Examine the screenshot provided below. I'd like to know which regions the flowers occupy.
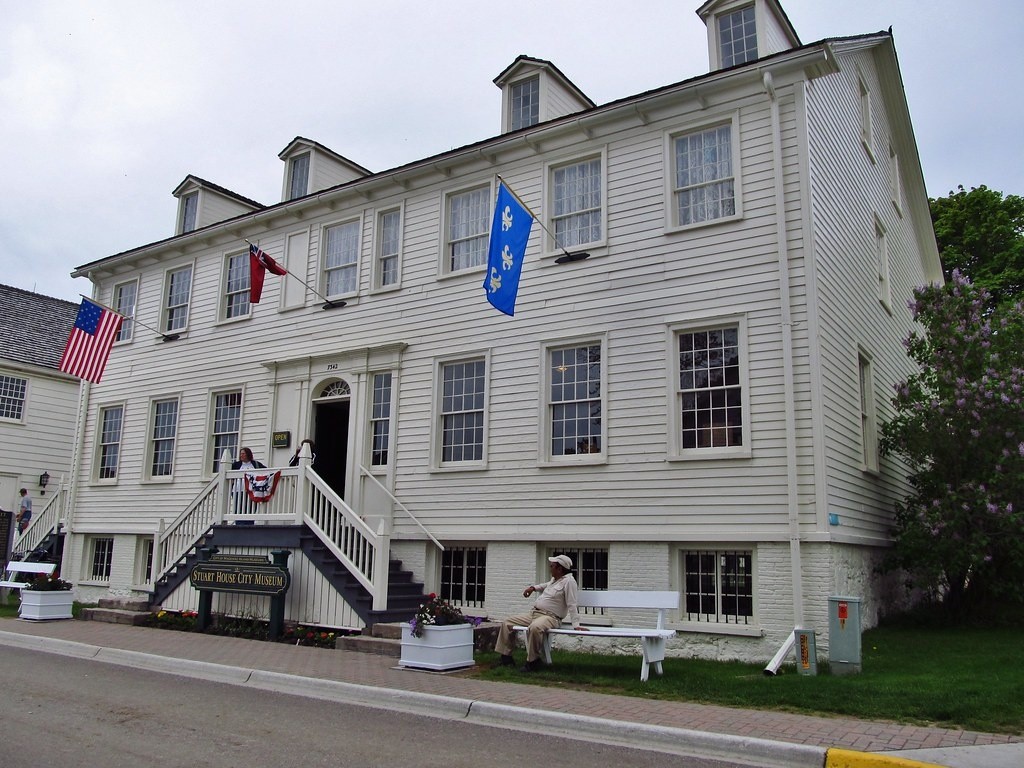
[409,592,483,640]
[24,572,74,591]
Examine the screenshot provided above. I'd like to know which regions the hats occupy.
[548,555,573,569]
[19,488,27,493]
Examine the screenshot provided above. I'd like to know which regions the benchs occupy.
[2,561,57,613]
[511,589,681,683]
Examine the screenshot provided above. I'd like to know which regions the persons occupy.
[15,488,32,535]
[19,518,29,533]
[229,447,270,524]
[488,553,590,673]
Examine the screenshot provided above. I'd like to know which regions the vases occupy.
[397,622,476,671]
[18,589,76,621]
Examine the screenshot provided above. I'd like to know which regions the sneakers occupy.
[519,657,543,672]
[501,654,515,668]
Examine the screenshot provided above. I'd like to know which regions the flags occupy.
[248,243,287,304]
[482,183,533,317]
[57,298,124,384]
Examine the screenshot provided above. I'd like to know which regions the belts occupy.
[533,606,563,620]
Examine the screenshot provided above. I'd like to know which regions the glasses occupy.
[549,564,558,568]
